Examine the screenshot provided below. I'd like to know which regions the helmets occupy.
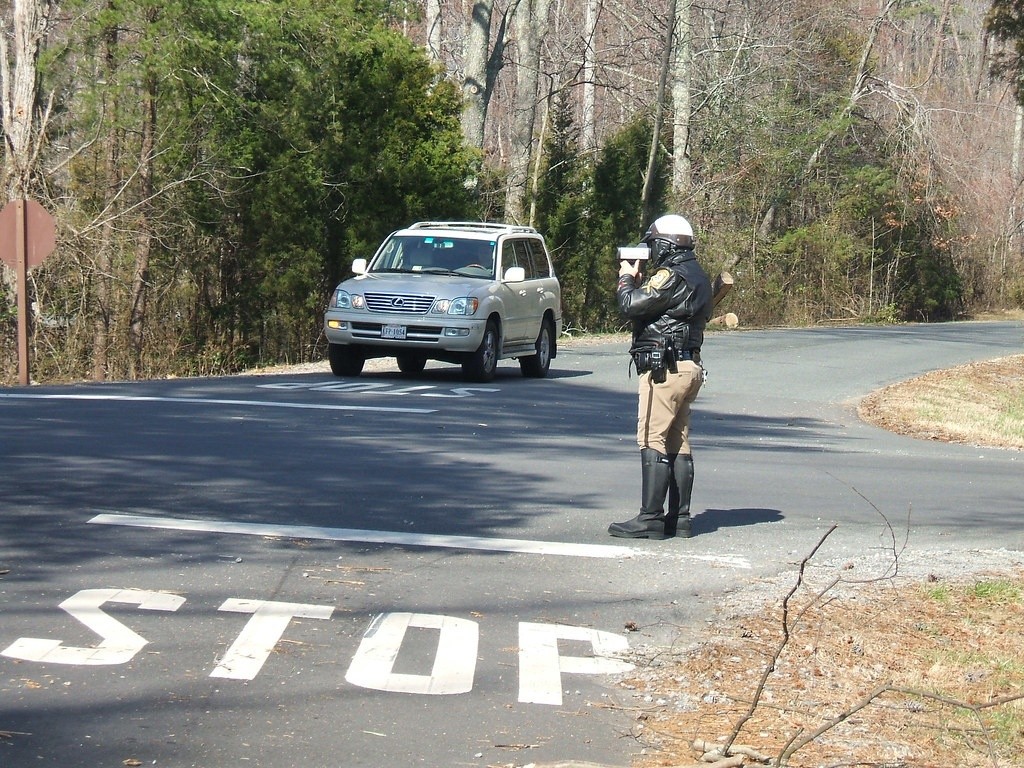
[637,215,697,251]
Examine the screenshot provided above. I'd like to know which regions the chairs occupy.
[403,248,434,270]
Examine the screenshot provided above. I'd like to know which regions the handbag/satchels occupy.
[628,331,680,385]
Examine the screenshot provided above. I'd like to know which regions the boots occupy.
[665,452,694,538]
[607,447,671,540]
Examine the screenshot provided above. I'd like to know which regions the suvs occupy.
[324,222,562,383]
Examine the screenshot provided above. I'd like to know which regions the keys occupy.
[701,369,708,388]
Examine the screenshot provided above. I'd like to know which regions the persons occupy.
[607,214,713,540]
